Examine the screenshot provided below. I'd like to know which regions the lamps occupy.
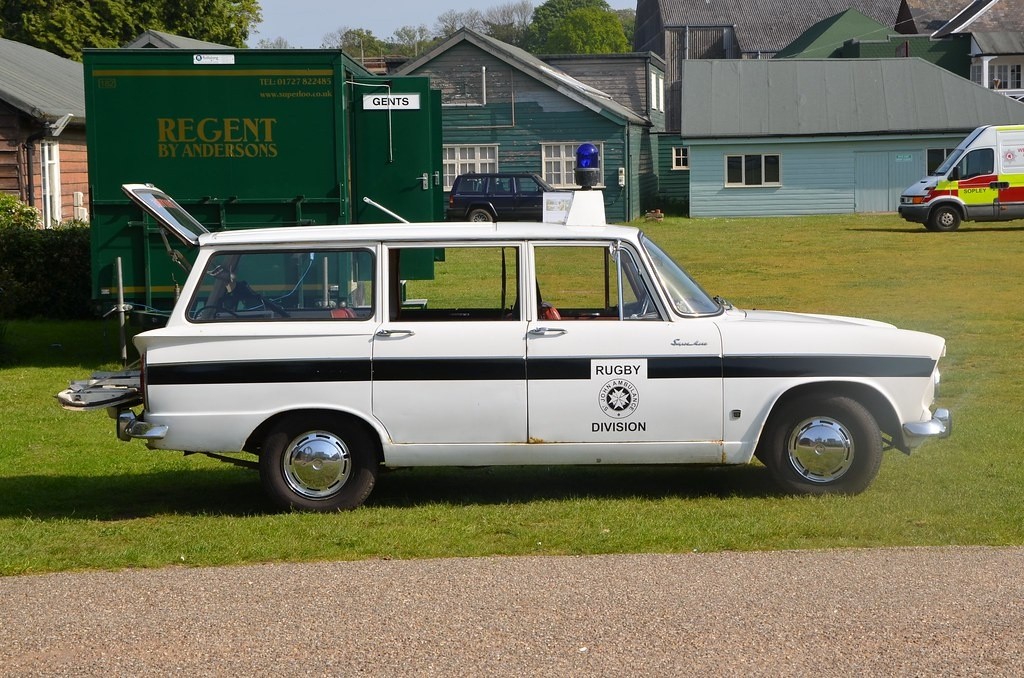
[49,113,75,137]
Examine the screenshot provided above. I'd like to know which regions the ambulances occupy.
[898,124,1024,231]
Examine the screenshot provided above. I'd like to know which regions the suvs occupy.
[447,170,566,221]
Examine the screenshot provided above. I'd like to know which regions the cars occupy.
[54,143,952,514]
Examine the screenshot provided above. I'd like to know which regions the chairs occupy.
[340,308,357,319]
[331,309,348,318]
[463,179,518,194]
[400,280,428,309]
[536,277,561,319]
